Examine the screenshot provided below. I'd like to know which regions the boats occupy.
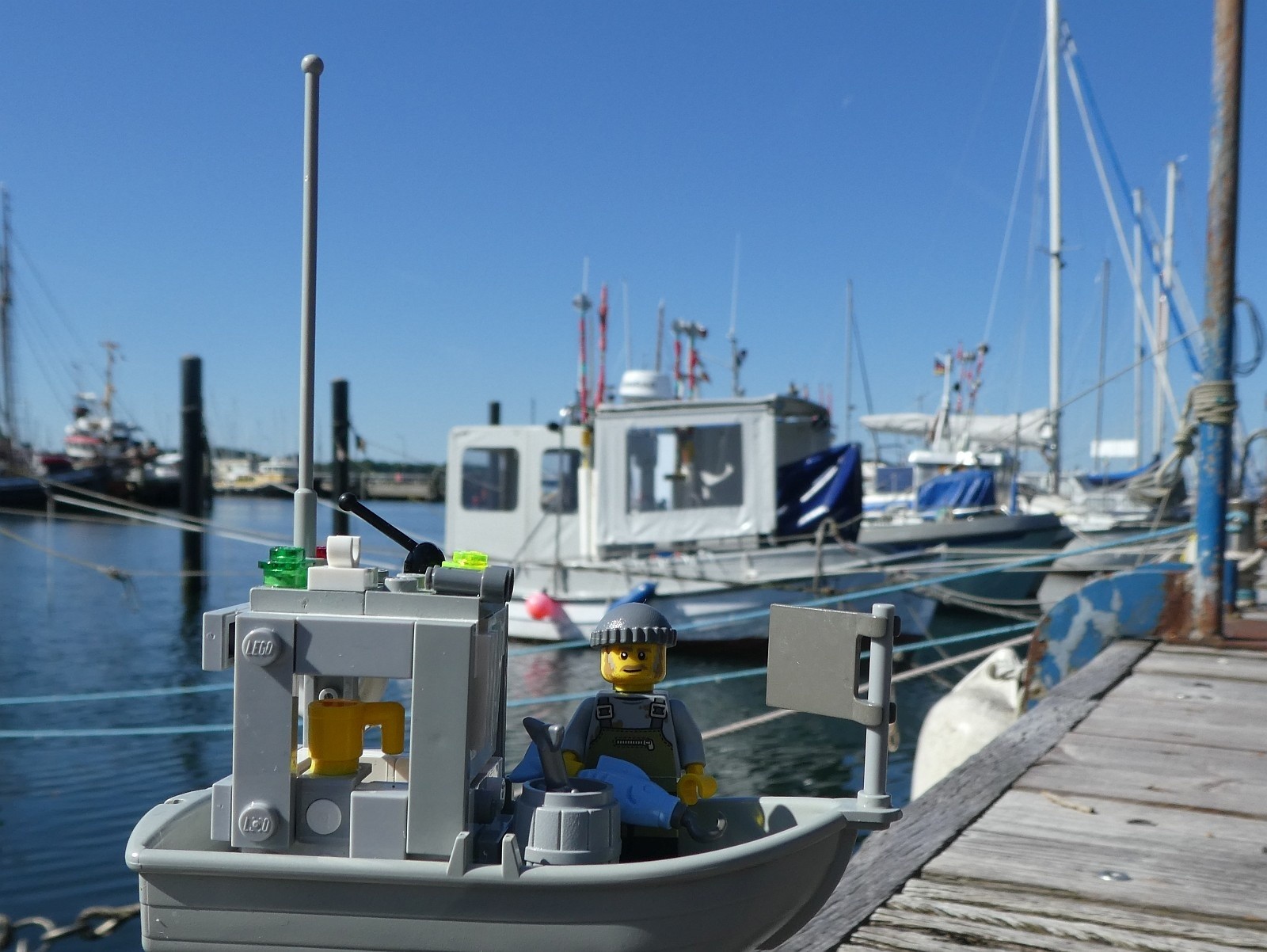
[424,265,1062,656]
[120,38,901,949]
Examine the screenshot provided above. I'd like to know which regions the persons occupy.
[561,602,718,840]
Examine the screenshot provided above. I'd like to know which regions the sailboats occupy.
[857,0,1251,611]
[0,200,182,515]
